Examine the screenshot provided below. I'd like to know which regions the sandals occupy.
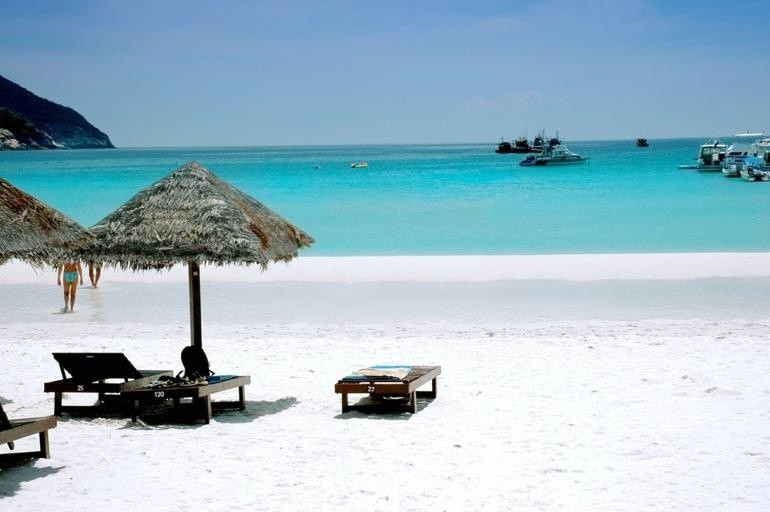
[162,371,208,387]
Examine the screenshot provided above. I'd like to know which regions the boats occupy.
[497,134,558,154]
[520,144,587,167]
[637,138,649,147]
[679,139,770,181]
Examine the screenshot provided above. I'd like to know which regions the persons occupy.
[88,261,103,288]
[57,257,83,313]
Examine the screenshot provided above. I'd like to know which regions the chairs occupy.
[0,404,57,458]
[44,353,173,417]
[335,364,442,414]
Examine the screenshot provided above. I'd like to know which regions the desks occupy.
[120,375,250,423]
